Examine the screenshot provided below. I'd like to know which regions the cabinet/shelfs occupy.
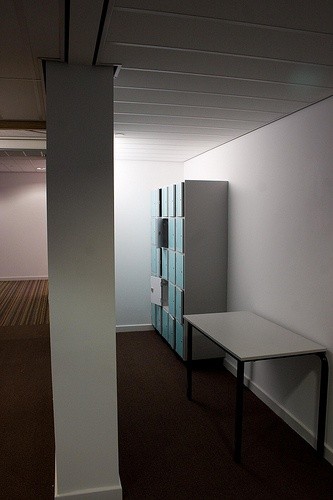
[150,180,228,368]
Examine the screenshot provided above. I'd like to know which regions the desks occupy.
[181,311,329,465]
